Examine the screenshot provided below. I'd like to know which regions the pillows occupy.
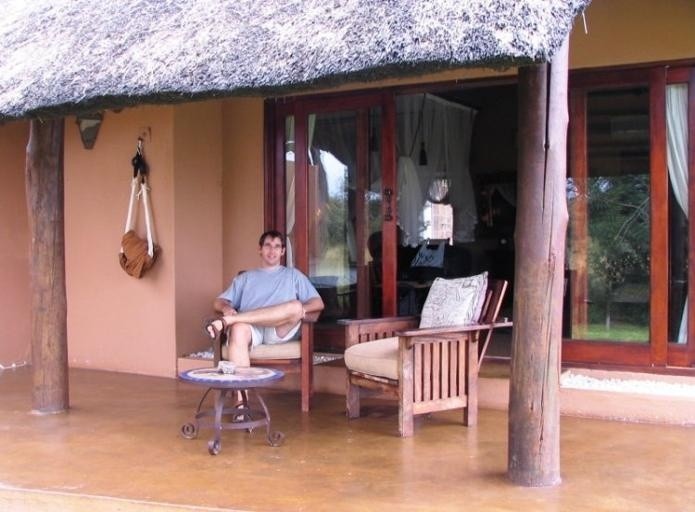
[417,272,489,330]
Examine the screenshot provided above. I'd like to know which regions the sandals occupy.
[232,401,255,431]
[203,314,227,339]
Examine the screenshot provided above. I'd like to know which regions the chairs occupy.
[208,314,315,415]
[338,271,512,440]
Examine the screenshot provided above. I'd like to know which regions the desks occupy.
[179,367,283,453]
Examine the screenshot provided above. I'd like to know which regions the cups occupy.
[223,365,234,373]
[218,361,229,372]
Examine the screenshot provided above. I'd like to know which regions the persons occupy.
[206,231,325,420]
